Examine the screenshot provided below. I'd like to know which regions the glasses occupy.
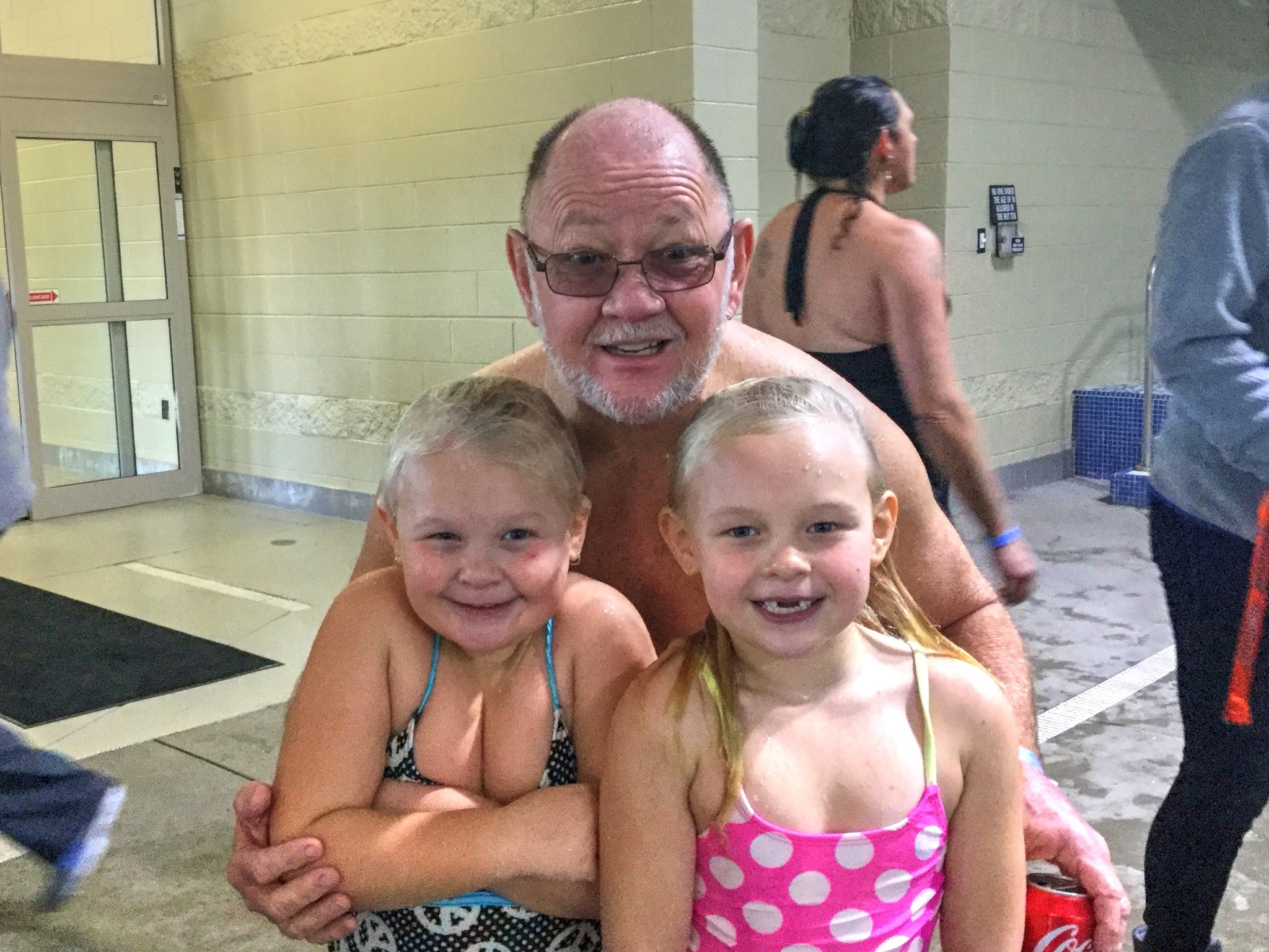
[524,218,736,297]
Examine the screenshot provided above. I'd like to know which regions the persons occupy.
[226,99,1130,952]
[0,291,125,911]
[1134,90,1268,952]
[744,76,1043,609]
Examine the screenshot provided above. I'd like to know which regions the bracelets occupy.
[989,526,1022,552]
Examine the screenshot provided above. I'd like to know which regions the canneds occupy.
[1021,872,1096,952]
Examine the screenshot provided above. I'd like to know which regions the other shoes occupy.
[43,773,127,910]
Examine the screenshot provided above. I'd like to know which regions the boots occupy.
[1132,923,1222,952]
